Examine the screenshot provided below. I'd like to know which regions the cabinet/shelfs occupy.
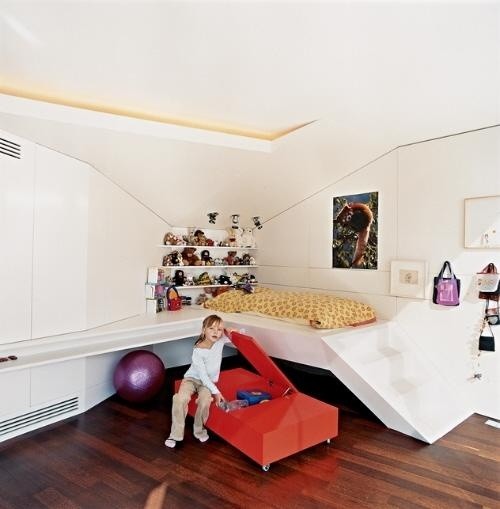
[175,331,338,472]
[159,245,258,301]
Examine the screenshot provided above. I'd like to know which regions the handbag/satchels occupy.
[476,263,500,292]
[437,263,459,306]
[479,318,495,352]
[480,261,500,295]
[486,314,500,326]
[434,261,460,303]
[479,267,500,301]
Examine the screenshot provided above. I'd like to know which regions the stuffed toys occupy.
[153,226,259,313]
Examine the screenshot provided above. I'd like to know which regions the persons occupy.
[164,315,245,449]
